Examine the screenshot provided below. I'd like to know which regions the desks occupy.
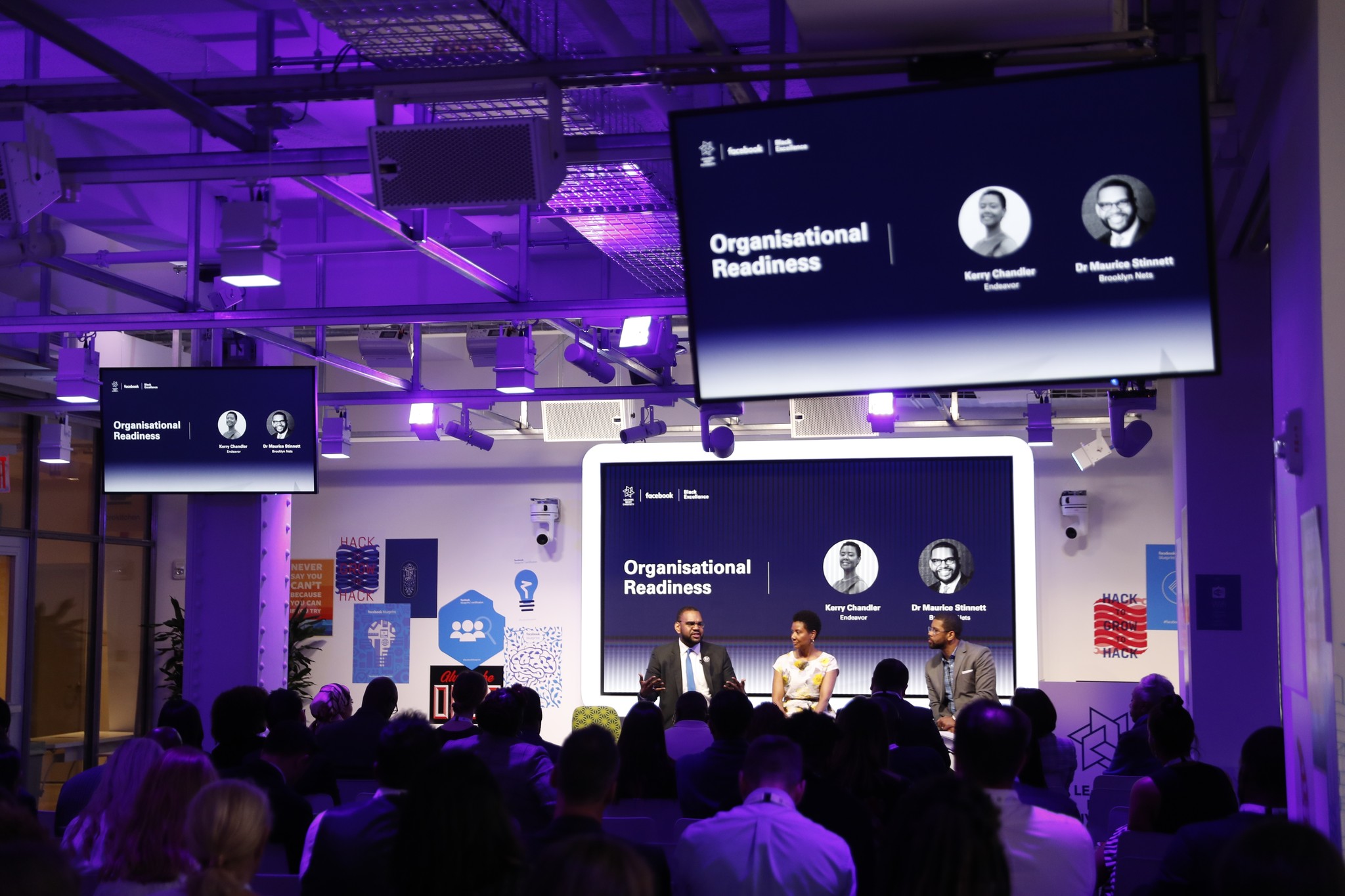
[32,729,133,795]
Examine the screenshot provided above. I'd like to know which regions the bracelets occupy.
[952,715,956,722]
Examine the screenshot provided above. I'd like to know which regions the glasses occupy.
[930,557,960,565]
[1097,198,1131,211]
[302,709,306,714]
[391,703,398,714]
[676,620,705,628]
[927,626,950,635]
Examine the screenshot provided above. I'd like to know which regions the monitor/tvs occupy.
[100,365,320,495]
[669,48,1224,406]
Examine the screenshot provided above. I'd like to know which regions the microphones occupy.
[700,659,704,664]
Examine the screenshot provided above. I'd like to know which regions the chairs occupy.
[608,815,657,847]
[251,778,378,896]
[1015,772,1180,896]
[571,707,622,743]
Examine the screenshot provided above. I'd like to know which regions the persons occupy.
[924,612,1000,734]
[0,669,1345,896]
[222,412,241,439]
[772,611,839,718]
[972,189,1021,258]
[926,542,972,594]
[833,542,869,594]
[637,607,747,729]
[1096,179,1152,254]
[271,412,293,439]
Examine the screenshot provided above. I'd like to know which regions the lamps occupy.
[1022,389,1057,447]
[619,405,667,444]
[1071,429,1113,470]
[491,323,538,396]
[618,314,679,371]
[563,321,615,384]
[218,181,285,287]
[37,412,74,465]
[407,403,443,442]
[698,401,744,458]
[444,406,496,453]
[1109,391,1157,457]
[53,333,101,404]
[320,403,352,460]
[867,392,900,435]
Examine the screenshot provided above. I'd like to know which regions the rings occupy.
[941,727,944,729]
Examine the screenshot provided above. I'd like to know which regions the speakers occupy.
[367,114,569,211]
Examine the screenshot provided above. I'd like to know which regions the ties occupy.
[686,649,696,692]
